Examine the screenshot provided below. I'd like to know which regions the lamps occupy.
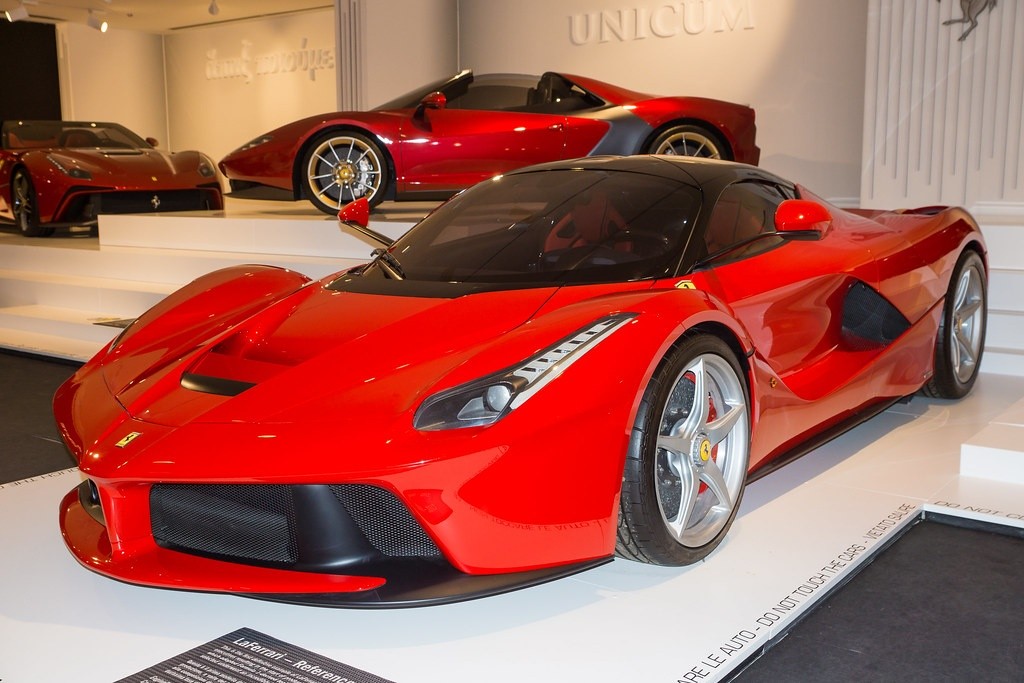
[5,3,30,23]
[87,14,109,33]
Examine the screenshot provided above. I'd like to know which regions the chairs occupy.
[704,201,771,260]
[539,196,634,256]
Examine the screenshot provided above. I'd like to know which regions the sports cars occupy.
[218,69,760,215]
[53,154,989,609]
[0,120,225,236]
